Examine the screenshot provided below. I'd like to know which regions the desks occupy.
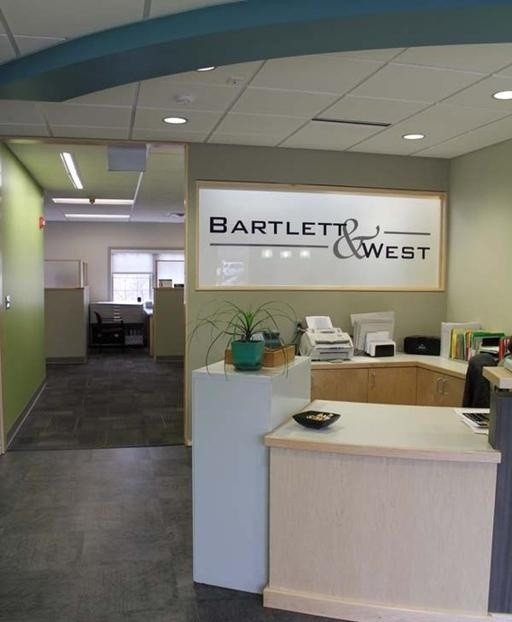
[143,308,154,356]
[262,398,502,621]
[481,365,511,621]
[191,353,312,595]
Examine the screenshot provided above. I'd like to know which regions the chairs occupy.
[93,310,125,354]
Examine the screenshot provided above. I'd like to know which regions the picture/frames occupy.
[193,179,448,291]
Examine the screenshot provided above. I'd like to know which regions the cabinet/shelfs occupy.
[311,369,368,405]
[416,367,466,404]
[44,286,89,365]
[152,286,185,360]
[368,367,416,405]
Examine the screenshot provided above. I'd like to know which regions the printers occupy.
[298,315,354,360]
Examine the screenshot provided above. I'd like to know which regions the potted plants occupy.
[186,297,305,381]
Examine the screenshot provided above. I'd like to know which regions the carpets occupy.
[4,352,184,452]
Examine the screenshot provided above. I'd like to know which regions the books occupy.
[451,328,512,362]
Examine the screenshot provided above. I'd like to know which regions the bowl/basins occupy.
[291,410,341,429]
